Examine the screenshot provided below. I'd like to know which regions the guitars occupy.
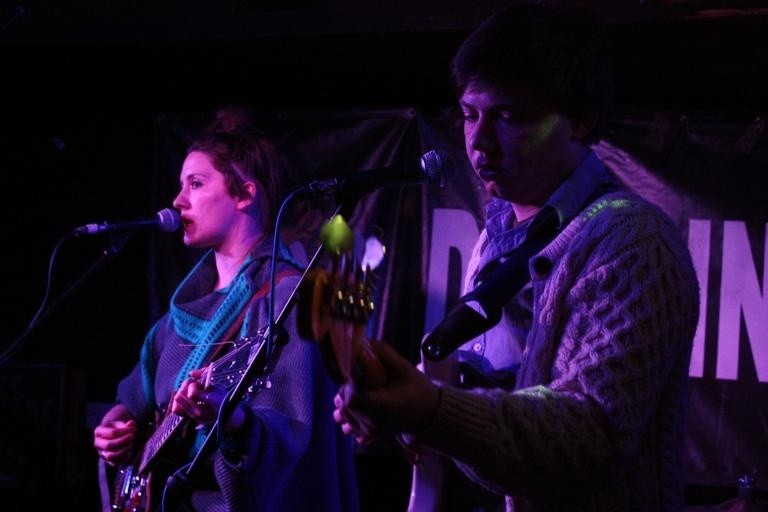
[109,323,286,511]
[296,254,458,512]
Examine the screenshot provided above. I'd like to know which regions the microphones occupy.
[293,148,453,204]
[76,206,182,238]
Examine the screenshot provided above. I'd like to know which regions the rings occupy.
[195,391,209,409]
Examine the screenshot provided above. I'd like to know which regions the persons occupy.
[89,103,364,511]
[331,10,704,510]
